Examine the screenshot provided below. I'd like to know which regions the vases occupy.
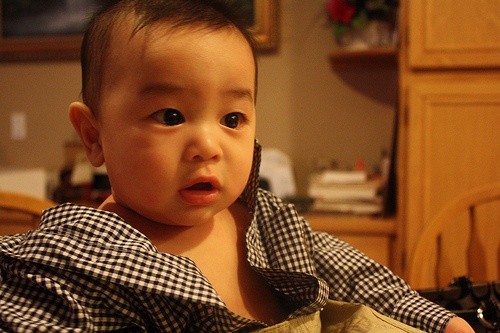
[346,18,389,48]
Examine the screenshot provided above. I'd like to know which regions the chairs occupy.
[407,182,500,290]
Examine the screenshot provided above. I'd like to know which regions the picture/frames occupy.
[0,0,280,63]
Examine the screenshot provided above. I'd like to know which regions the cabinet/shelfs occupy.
[398,0,500,290]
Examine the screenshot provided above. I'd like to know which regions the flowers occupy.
[322,0,376,48]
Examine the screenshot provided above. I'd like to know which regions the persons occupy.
[1,0,476,333]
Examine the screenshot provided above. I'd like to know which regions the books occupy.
[309,168,383,215]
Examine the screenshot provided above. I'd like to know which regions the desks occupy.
[52,194,396,285]
